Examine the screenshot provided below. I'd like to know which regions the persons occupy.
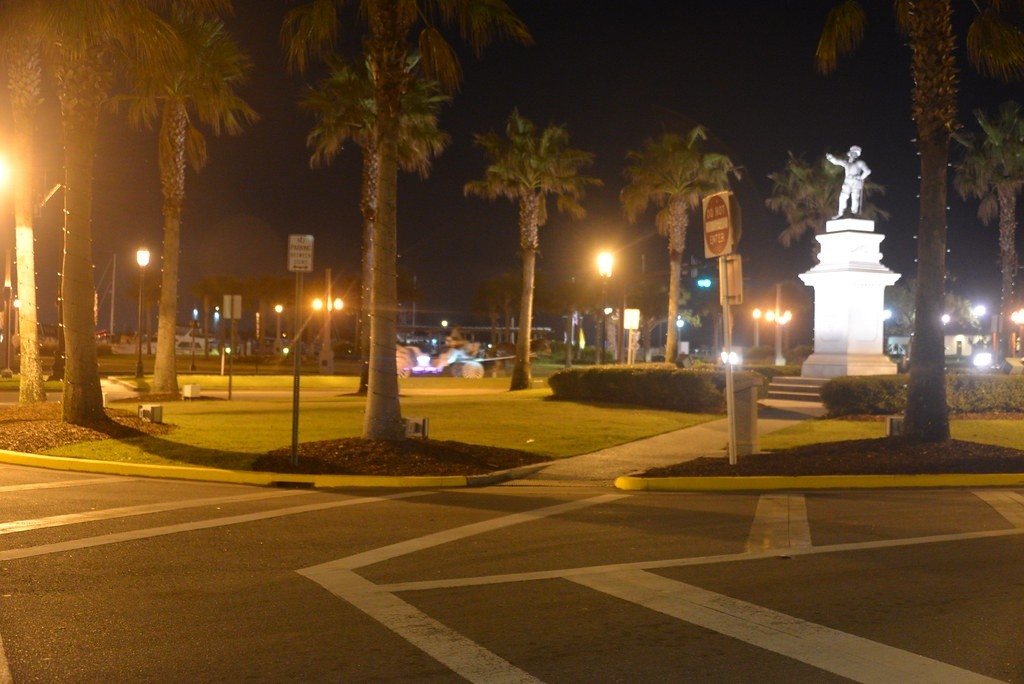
[825,145,871,220]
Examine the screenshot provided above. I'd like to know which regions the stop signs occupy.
[701,191,734,258]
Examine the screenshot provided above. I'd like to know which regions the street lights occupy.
[275,300,284,355]
[312,295,348,376]
[596,248,617,365]
[750,301,1024,381]
[441,319,448,352]
[190,304,198,374]
[135,244,151,379]
[212,303,220,358]
